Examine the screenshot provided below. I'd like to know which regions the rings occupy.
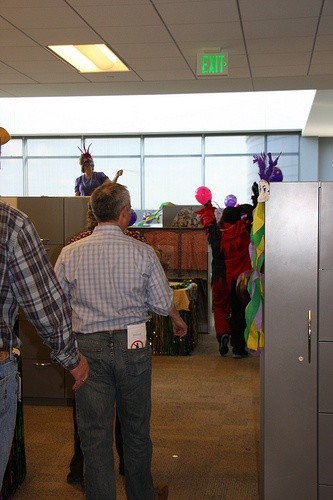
[82,376,87,382]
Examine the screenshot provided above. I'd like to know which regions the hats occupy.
[81,154,92,173]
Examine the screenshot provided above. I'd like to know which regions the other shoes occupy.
[154,485,169,500]
[66,471,87,491]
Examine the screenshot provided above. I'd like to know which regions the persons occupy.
[64,198,188,488]
[198,181,260,361]
[74,153,123,197]
[0,126,91,491]
[52,180,176,499]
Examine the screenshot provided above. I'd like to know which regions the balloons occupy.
[223,194,237,209]
[264,166,284,182]
[127,209,137,226]
[194,186,213,205]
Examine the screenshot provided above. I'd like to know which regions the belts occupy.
[0,348,21,361]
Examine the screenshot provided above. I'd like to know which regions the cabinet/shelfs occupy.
[127,228,210,281]
[2,195,95,407]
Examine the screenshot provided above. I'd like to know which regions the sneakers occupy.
[220,333,230,354]
[232,347,249,359]
